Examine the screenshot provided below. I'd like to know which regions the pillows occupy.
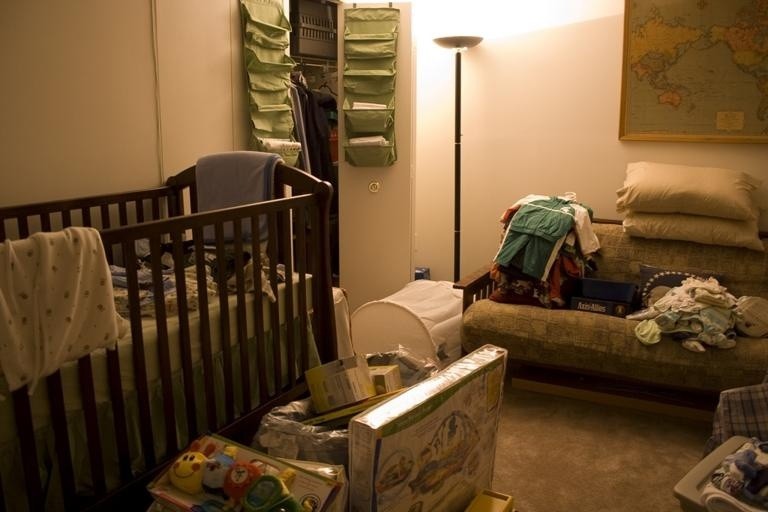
[617,161,765,252]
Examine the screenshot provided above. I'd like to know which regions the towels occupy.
[194,151,284,246]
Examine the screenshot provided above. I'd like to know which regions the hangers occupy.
[317,64,337,96]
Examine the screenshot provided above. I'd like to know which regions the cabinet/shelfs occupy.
[230,0,414,316]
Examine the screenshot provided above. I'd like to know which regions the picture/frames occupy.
[617,0,767,146]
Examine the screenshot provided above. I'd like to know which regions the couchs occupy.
[453,218,768,389]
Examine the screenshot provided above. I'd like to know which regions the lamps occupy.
[434,36,482,283]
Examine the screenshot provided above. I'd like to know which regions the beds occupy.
[1,150,338,511]
